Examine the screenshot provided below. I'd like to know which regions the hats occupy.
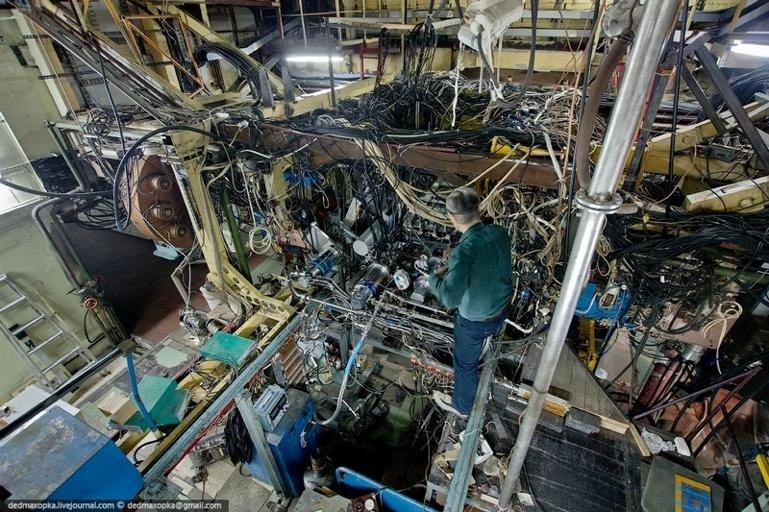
[446,187,479,215]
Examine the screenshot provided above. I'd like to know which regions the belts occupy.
[488,304,509,322]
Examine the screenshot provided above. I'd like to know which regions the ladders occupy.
[0,274,110,392]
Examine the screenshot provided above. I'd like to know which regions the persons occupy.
[429,188,511,418]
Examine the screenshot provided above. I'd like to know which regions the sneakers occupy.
[479,335,491,360]
[434,390,469,420]
